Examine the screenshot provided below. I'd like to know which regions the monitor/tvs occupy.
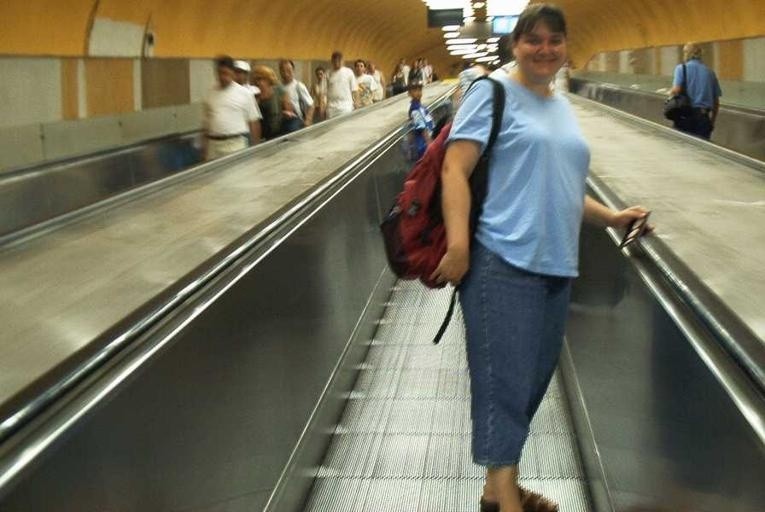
[493,15,518,35]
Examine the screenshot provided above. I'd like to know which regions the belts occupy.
[204,132,249,141]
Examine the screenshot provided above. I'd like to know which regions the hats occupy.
[232,60,251,72]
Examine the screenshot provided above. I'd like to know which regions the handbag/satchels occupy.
[663,91,692,120]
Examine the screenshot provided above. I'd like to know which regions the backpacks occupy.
[379,73,507,288]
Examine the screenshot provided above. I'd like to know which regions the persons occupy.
[426,4,653,511]
[555,66,569,92]
[454,58,484,96]
[666,43,721,141]
[197,52,434,162]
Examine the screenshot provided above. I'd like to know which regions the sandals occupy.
[481,482,558,512]
